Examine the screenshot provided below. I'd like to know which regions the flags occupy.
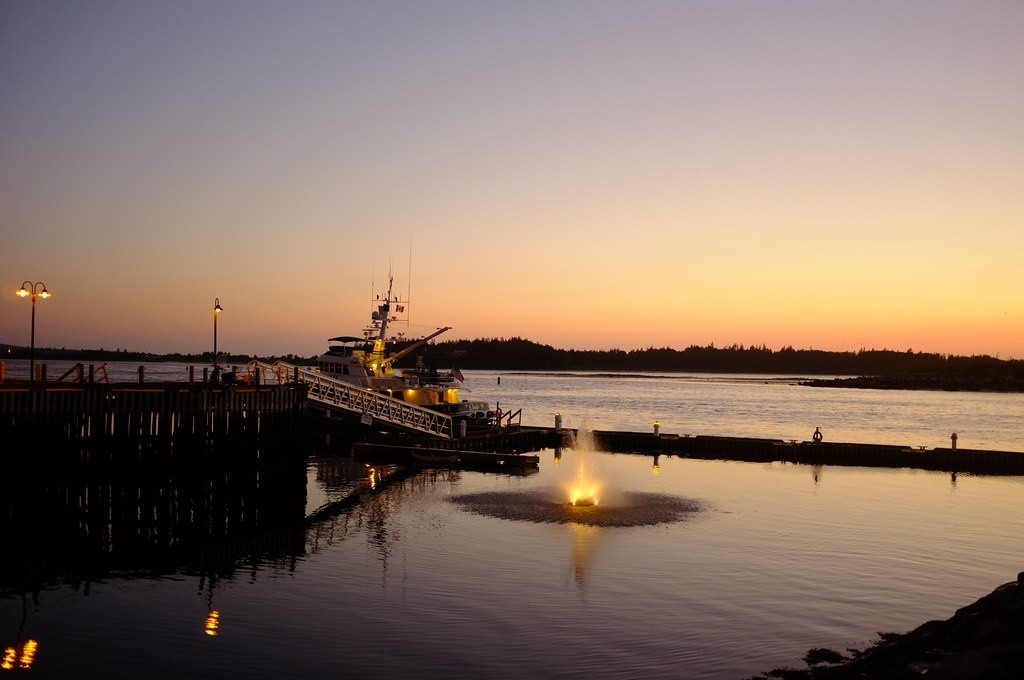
[450,367,464,383]
[396,305,404,313]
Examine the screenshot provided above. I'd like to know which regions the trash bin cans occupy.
[555,413,562,430]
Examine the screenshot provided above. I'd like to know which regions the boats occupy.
[289,241,504,424]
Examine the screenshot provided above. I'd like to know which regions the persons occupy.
[382,366,386,373]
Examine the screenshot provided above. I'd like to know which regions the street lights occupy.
[16,281,51,382]
[213,298,223,362]
